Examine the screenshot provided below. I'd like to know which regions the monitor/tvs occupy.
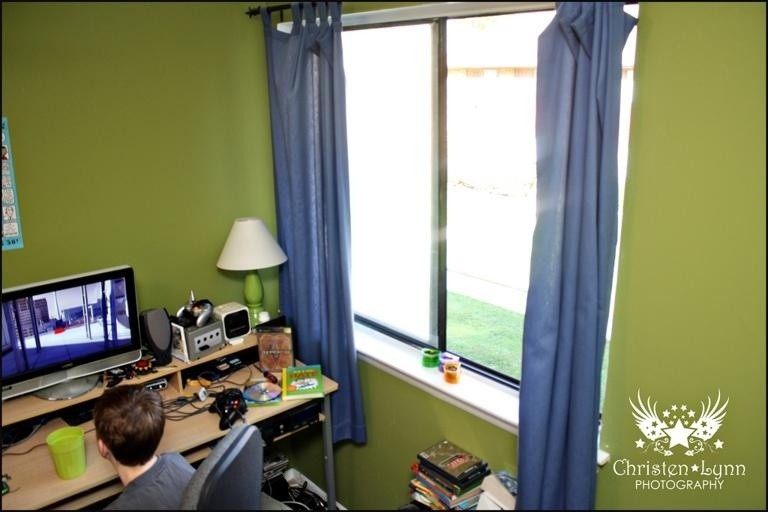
[2,264,142,400]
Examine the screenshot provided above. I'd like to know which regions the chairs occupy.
[175,422,266,511]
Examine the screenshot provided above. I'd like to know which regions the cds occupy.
[248,382,282,402]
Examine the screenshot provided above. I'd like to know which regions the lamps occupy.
[215,217,288,313]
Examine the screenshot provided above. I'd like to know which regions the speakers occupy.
[139,307,173,367]
[213,301,252,346]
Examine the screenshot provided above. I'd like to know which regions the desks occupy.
[1,332,340,510]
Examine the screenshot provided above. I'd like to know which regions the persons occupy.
[89,384,197,511]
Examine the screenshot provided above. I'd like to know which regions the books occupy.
[408,439,491,511]
[281,364,325,399]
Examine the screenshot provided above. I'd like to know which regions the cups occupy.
[46,426,87,480]
